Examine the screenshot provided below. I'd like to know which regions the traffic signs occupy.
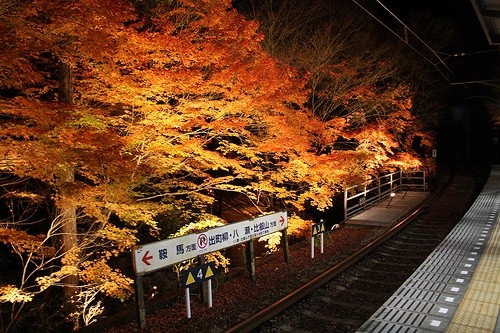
[123,209,289,279]
